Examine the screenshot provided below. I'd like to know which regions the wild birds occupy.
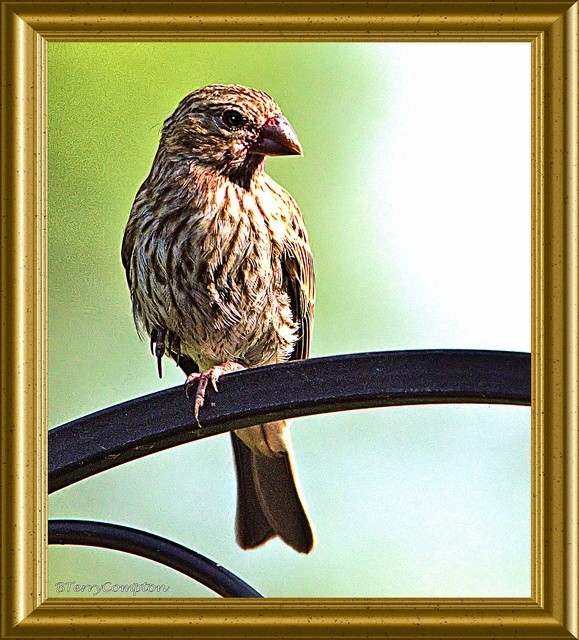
[120,83,314,554]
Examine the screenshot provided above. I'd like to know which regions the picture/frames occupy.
[1,1,579,640]
